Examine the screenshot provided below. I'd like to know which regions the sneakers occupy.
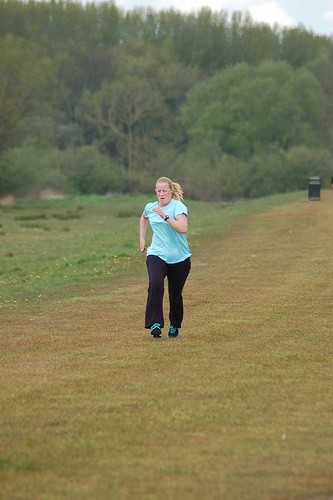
[150,322,161,338]
[167,322,179,338]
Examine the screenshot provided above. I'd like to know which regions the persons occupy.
[138,176,192,338]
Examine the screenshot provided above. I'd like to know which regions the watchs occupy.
[163,215,170,220]
